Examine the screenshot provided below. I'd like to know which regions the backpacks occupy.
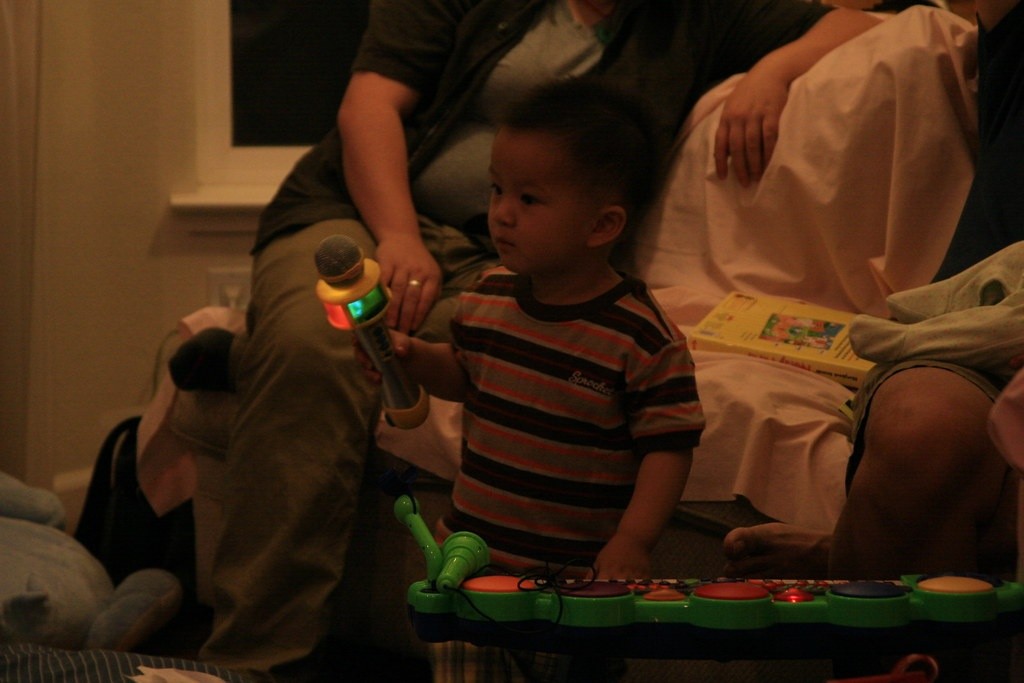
[79,420,199,595]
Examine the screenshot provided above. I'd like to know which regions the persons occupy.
[719,1,1024,579]
[349,74,706,683]
[169,0,882,683]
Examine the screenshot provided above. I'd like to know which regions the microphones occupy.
[316,234,429,430]
[435,531,491,594]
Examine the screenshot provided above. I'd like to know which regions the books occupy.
[688,290,878,389]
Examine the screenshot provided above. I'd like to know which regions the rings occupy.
[409,280,421,286]
